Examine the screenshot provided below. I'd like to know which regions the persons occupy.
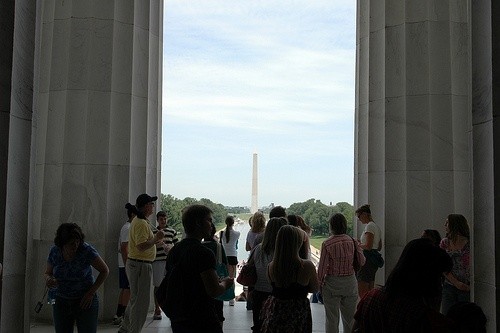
[46,222,109,333]
[112,194,486,333]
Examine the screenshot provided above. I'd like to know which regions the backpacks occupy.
[447,303,486,333]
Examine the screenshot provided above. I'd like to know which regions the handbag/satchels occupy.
[351,236,361,273]
[156,269,187,317]
[237,245,258,286]
[227,256,238,265]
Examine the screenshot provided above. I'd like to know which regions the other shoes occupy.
[118,325,129,333]
[112,314,124,326]
[229,300,234,306]
[154,309,161,319]
[238,291,248,301]
[317,292,325,304]
[312,294,318,303]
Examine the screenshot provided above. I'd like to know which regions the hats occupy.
[136,194,157,206]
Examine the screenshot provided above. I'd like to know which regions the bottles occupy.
[47,277,56,304]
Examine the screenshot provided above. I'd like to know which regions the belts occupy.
[127,257,153,264]
